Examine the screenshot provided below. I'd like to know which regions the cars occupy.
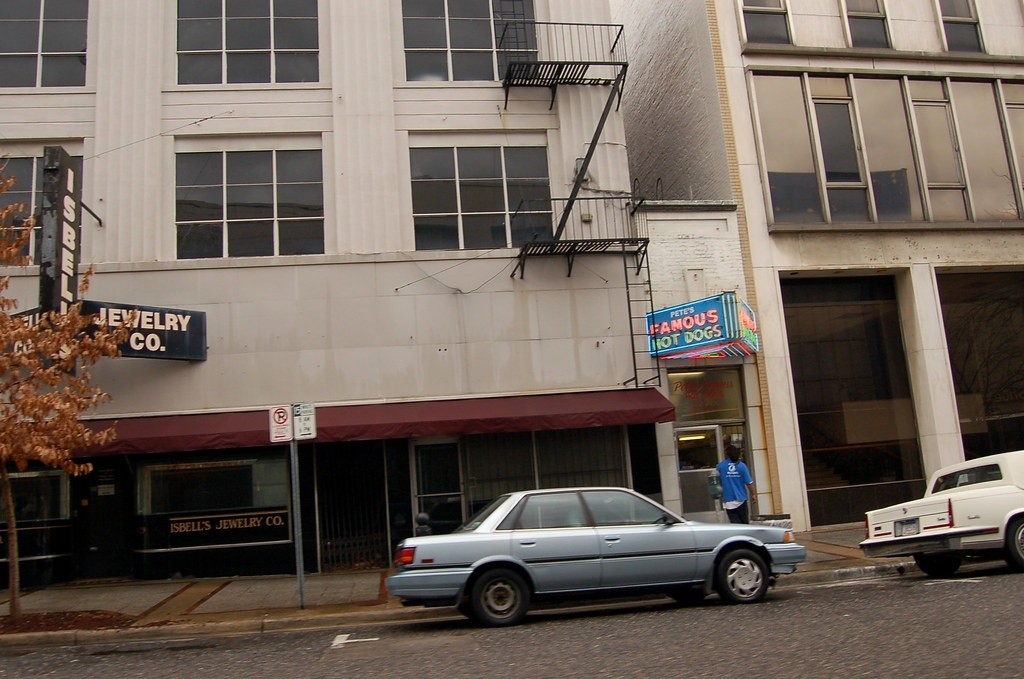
[389,486,808,627]
[860,450,1024,575]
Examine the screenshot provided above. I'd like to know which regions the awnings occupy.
[32,387,676,459]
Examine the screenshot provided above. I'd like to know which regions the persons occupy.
[716,444,756,524]
